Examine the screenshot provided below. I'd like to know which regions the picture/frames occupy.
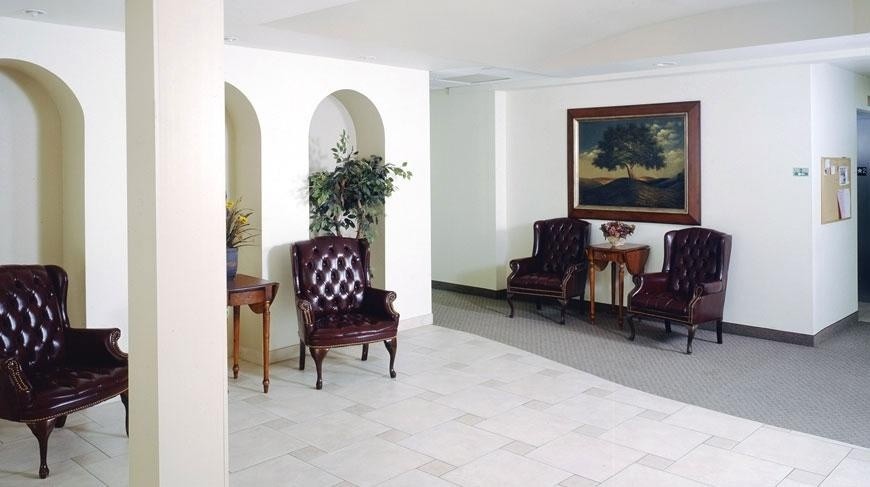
[566,100,701,225]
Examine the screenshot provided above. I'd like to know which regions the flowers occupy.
[226,190,263,249]
[599,221,635,240]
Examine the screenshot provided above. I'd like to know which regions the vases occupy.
[227,247,239,280]
[608,236,621,247]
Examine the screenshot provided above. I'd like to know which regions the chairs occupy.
[625,227,732,354]
[291,235,400,390]
[507,217,591,325]
[0,265,129,478]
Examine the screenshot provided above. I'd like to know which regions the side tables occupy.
[587,242,650,330]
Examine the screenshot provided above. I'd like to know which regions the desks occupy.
[227,274,280,394]
